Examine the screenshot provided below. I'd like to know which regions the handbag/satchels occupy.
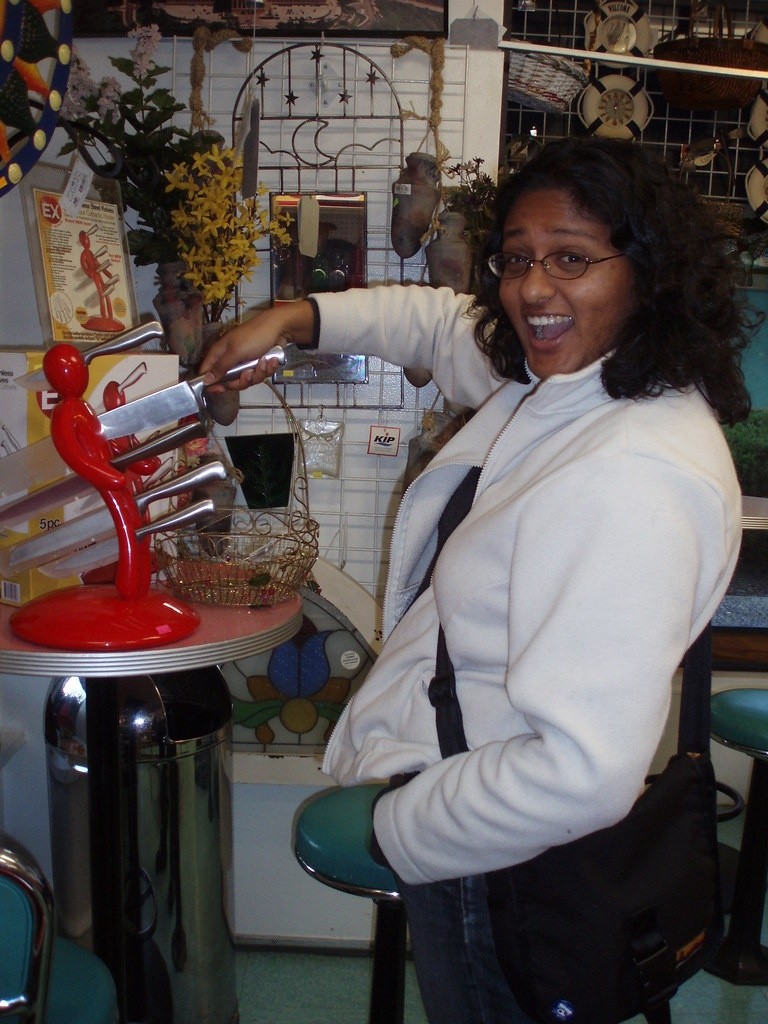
[486,752,725,1024]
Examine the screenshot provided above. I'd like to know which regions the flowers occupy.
[446,157,502,263]
[167,141,295,322]
[53,19,233,268]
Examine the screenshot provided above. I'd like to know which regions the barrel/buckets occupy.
[223,432,298,510]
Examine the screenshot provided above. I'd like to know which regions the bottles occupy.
[312,248,329,286]
[330,250,353,285]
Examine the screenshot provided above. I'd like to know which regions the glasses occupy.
[488,251,626,280]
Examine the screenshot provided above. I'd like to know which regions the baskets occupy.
[154,378,319,607]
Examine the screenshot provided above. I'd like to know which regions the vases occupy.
[199,319,240,425]
[425,208,473,295]
[153,288,203,367]
[391,151,443,260]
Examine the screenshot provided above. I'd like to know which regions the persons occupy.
[196,134,744,1023]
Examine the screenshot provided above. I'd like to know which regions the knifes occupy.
[0,422,207,522]
[38,499,216,578]
[9,461,226,568]
[13,321,164,392]
[97,345,287,441]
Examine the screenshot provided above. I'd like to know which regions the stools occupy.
[291,779,415,1024]
[686,687,768,986]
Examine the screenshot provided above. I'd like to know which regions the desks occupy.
[0,579,304,1024]
